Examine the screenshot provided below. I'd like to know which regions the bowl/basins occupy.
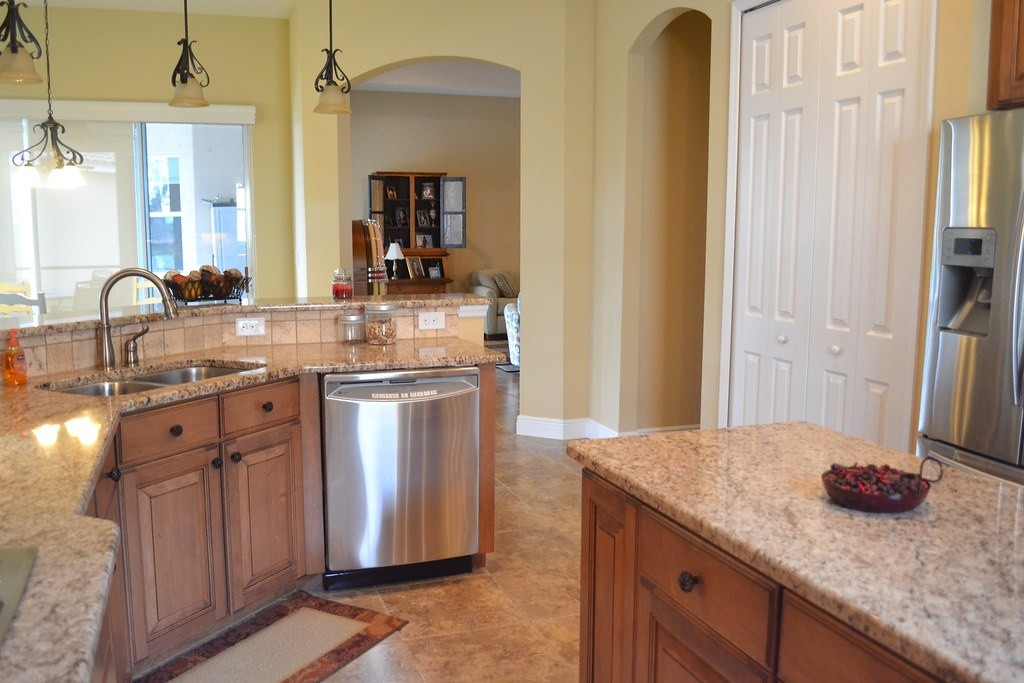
[822,467,930,513]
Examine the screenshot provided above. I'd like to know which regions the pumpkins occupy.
[172,273,234,301]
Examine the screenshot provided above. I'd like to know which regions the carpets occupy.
[496,364,520,372]
[132,590,409,683]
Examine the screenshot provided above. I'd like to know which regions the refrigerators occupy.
[915,107,1024,486]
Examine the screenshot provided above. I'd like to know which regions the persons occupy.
[397,209,408,226]
[411,260,422,276]
[417,236,429,248]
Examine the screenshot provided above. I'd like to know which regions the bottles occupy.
[338,314,365,345]
[364,303,397,345]
[332,268,353,302]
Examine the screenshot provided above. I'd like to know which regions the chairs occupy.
[0,280,106,316]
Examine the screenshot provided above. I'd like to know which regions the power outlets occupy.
[235,318,266,337]
[419,312,445,329]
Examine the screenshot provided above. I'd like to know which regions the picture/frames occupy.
[386,187,397,199]
[421,183,435,199]
[406,256,425,279]
[415,233,433,249]
[428,267,441,278]
[416,210,429,227]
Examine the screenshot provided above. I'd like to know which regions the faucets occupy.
[96,267,179,371]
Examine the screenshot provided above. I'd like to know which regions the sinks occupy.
[131,366,254,385]
[33,382,166,396]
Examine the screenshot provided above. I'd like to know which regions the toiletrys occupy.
[4,328,27,387]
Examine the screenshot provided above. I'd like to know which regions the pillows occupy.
[496,271,518,298]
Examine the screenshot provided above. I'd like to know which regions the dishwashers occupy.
[317,365,479,591]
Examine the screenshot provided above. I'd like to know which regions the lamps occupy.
[169,0,210,108]
[13,0,84,188]
[313,0,352,115]
[385,243,405,280]
[0,0,43,84]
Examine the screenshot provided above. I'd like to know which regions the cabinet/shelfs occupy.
[368,172,466,293]
[85,376,306,683]
[987,0,1024,111]
[579,468,937,683]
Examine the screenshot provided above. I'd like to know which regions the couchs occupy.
[469,269,520,340]
[504,292,521,365]
